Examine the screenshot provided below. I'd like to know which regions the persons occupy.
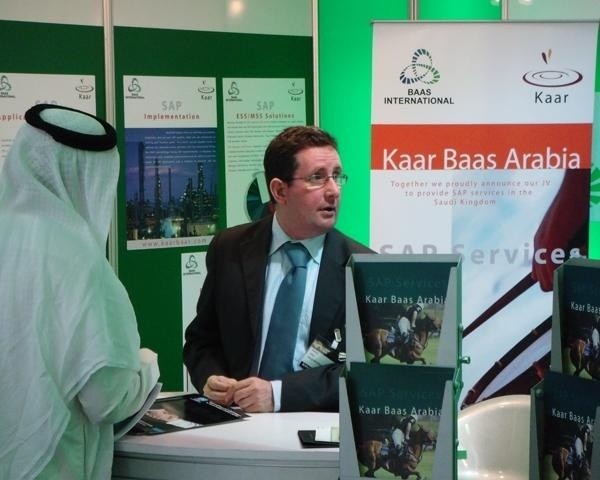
[180,124,375,412]
[573,419,593,474]
[393,300,425,355]
[389,413,419,468]
[0,102,162,480]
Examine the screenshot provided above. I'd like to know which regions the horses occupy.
[550,440,594,479]
[567,338,600,380]
[362,311,438,365]
[359,423,436,478]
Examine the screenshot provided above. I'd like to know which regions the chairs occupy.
[458,394,531,480]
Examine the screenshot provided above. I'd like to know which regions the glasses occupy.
[292,174,348,187]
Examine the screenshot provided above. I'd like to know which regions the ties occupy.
[257,241,313,380]
